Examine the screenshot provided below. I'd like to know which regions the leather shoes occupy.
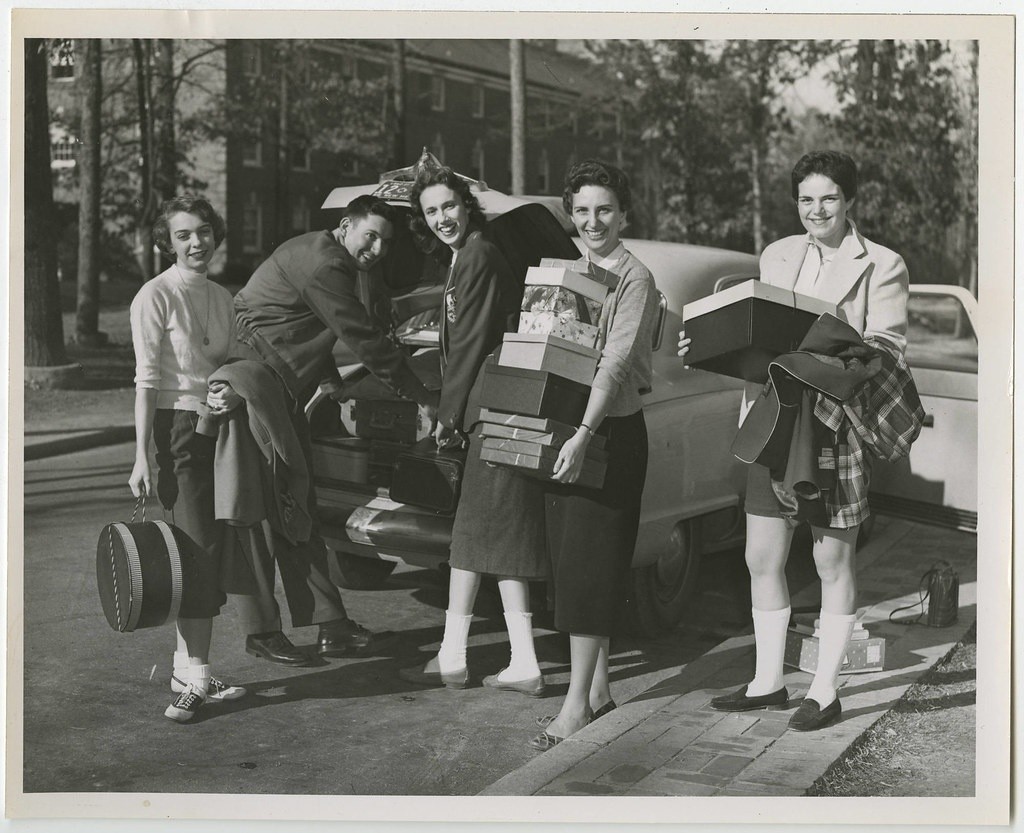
[710,685,789,712]
[318,619,387,657]
[396,659,470,689]
[246,631,312,666]
[787,694,842,731]
[478,668,545,698]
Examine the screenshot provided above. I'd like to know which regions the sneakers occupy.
[170,674,247,699]
[164,683,207,722]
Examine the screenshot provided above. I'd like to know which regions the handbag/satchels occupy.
[889,561,959,627]
[311,389,466,515]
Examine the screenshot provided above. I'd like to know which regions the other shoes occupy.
[527,708,598,752]
[535,700,617,729]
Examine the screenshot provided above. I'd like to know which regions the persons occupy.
[533,160,664,751]
[126,191,252,722]
[675,149,909,730]
[396,158,550,697]
[218,194,440,666]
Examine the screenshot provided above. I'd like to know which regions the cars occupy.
[305,146,980,649]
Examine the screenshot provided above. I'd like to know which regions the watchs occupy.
[580,423,595,441]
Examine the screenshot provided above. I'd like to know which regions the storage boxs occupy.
[498,332,602,387]
[482,435,607,478]
[478,407,608,451]
[539,257,620,291]
[524,267,610,304]
[479,448,606,492]
[783,629,886,677]
[477,364,612,437]
[481,422,611,464]
[520,286,603,327]
[517,311,600,348]
[682,279,838,386]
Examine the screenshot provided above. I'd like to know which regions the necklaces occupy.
[174,262,211,347]
